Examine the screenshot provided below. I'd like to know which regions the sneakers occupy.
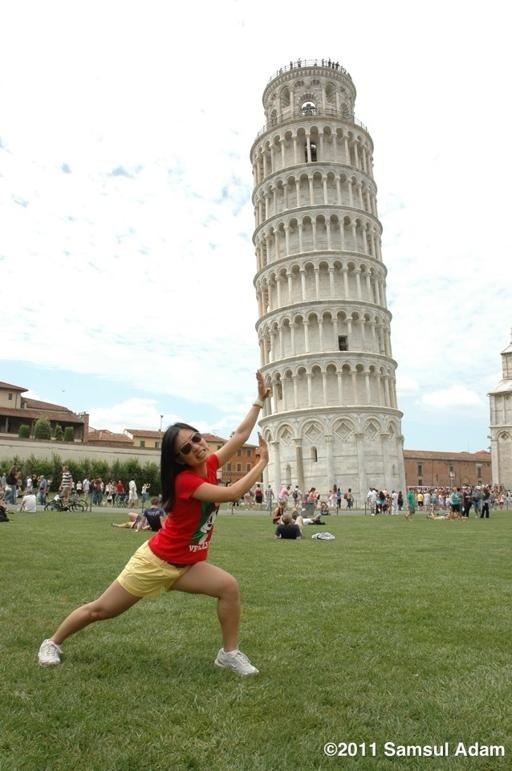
[215,648,259,678]
[38,640,61,669]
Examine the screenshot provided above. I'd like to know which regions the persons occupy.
[270,502,287,525]
[34,368,272,679]
[131,496,166,534]
[273,513,303,539]
[111,514,150,531]
[1,466,512,521]
[291,511,325,525]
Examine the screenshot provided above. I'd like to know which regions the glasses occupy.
[177,432,201,457]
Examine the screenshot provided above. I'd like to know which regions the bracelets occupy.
[252,400,264,409]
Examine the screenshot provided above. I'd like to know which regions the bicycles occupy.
[44,491,88,513]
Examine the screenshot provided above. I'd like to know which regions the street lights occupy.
[160,415,164,428]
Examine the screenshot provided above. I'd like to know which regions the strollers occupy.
[116,492,128,509]
